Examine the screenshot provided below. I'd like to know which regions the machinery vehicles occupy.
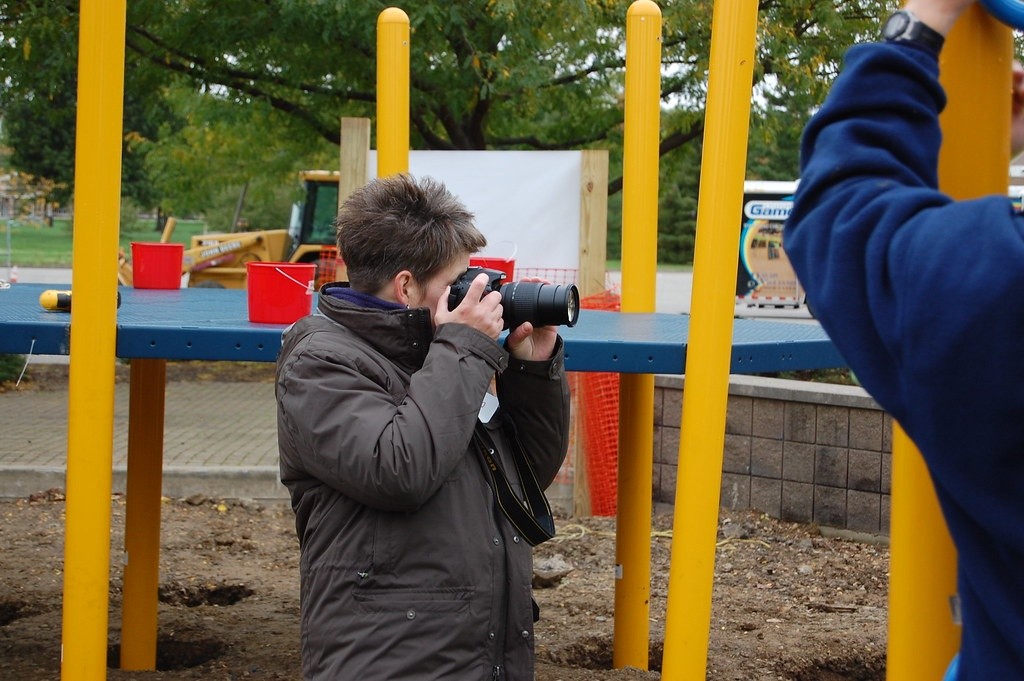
[118,170,340,289]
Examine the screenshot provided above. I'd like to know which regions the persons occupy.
[782,4,1024,681]
[275,179,571,681]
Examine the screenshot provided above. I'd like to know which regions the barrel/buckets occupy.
[245,261,318,324]
[130,242,184,290]
[470,241,517,287]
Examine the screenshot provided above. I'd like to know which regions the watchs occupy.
[880,11,944,59]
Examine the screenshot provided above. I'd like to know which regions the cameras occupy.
[448,265,580,330]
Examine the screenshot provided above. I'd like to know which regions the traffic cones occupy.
[10,264,18,283]
